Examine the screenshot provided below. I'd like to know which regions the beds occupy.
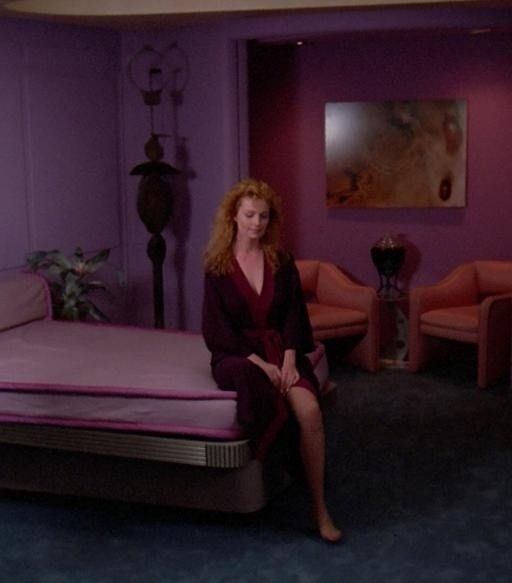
[0,270,296,513]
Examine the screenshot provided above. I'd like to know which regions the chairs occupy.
[294,258,380,372]
[409,259,512,387]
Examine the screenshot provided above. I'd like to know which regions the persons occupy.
[199,175,346,545]
